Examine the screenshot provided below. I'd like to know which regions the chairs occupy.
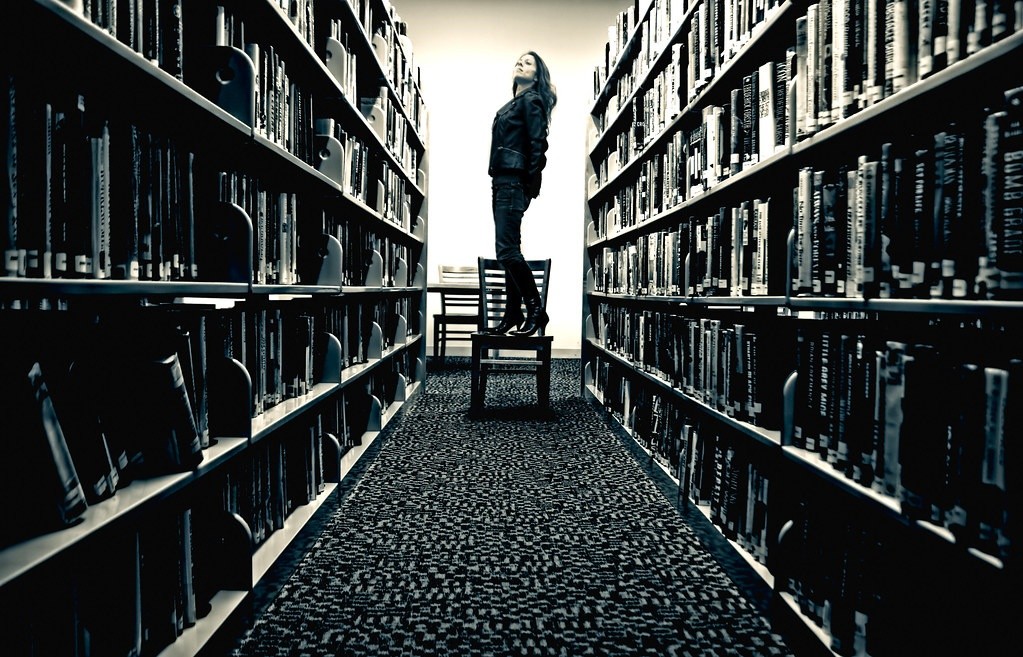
[434,262,496,358]
[469,255,553,414]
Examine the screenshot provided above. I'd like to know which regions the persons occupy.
[484,50,558,337]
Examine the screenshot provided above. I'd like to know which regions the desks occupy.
[427,284,504,359]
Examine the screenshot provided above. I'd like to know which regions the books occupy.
[0,0,428,657]
[585,0,1023,657]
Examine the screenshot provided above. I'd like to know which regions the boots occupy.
[482,305,526,334]
[510,298,549,337]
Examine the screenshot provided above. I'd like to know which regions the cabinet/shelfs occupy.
[1,1,441,656]
[576,1,1022,656]
